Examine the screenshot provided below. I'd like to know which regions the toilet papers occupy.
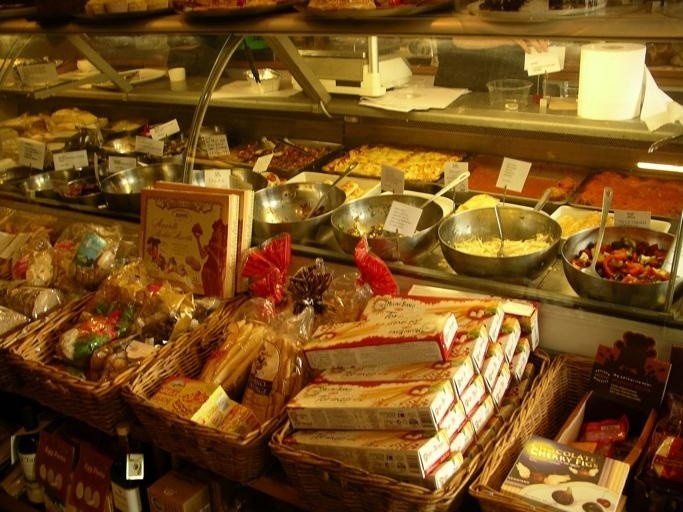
[576,42,683,132]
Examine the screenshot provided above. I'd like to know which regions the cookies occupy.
[5,285,64,319]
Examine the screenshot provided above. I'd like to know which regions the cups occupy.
[244,69,281,93]
[167,67,185,83]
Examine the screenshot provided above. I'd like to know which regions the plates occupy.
[293,1,452,22]
[68,1,293,20]
[92,68,165,90]
[467,0,607,23]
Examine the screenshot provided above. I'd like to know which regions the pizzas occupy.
[325,146,461,182]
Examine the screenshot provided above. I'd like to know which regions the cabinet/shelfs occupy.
[1,18,683,358]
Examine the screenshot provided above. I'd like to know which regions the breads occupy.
[197,267,363,418]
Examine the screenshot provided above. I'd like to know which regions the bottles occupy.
[15,409,45,508]
[108,422,151,512]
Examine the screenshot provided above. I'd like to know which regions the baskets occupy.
[122,245,324,485]
[264,261,554,511]
[2,215,248,443]
[468,310,672,512]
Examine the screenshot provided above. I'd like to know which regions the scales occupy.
[291,34,411,97]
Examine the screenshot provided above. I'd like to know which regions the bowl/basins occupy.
[0,153,268,212]
[245,180,346,246]
[328,194,443,267]
[438,204,562,285]
[559,224,683,306]
[485,78,532,108]
[100,119,144,141]
